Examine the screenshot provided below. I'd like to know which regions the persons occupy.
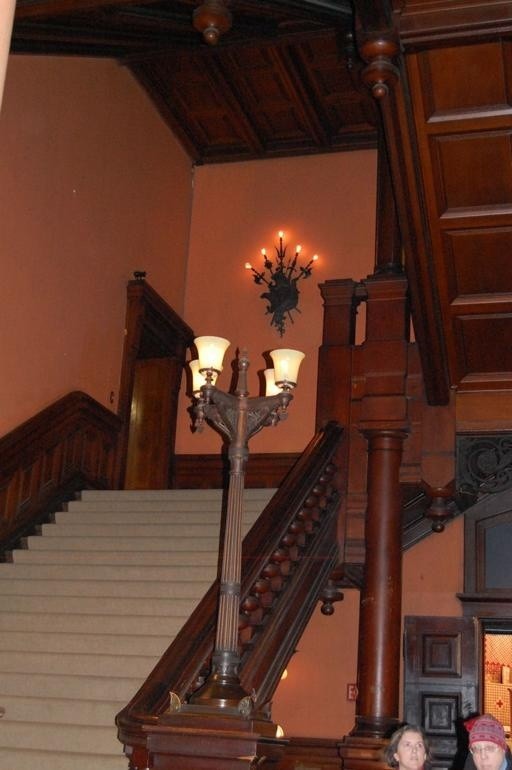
[380,723,438,770]
[458,711,512,770]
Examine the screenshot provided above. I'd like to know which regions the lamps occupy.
[166,335,306,719]
[245,230,319,338]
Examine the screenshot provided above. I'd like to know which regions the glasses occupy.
[469,743,500,754]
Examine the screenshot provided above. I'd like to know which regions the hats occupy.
[467,713,508,751]
